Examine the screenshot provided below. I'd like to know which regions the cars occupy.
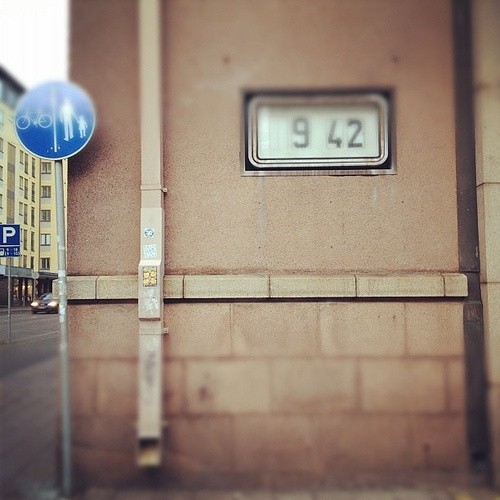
[30,292,59,314]
